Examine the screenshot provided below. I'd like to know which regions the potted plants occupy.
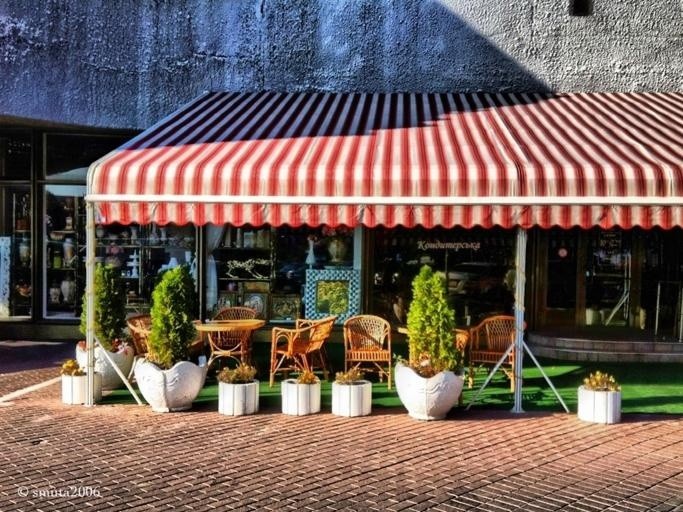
[75,263,135,392]
[281,353,322,416]
[331,361,373,419]
[61,360,103,404]
[577,370,622,424]
[394,265,466,422]
[133,263,208,412]
[216,362,260,416]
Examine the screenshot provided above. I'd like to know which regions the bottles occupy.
[53,251,62,269]
[18,237,30,267]
[63,237,74,268]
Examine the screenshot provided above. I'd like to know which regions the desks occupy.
[196,319,266,369]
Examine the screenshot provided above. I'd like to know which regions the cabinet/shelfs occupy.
[1,183,35,326]
[34,180,202,325]
[199,223,361,320]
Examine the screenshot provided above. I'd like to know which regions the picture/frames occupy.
[241,289,269,321]
[210,291,238,318]
[217,278,270,305]
[305,268,361,325]
[270,294,302,321]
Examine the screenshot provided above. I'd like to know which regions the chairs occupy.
[268,315,339,386]
[207,307,256,375]
[343,315,392,389]
[126,315,205,384]
[420,328,470,404]
[468,316,528,392]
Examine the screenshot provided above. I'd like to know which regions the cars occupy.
[272,258,324,294]
[447,250,511,308]
